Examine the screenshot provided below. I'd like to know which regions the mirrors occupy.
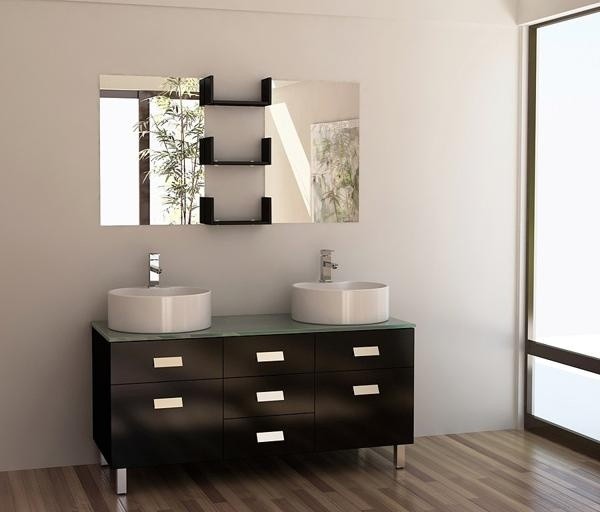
[264,80,359,224]
[99,74,205,225]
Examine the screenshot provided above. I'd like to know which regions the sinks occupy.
[291,281,390,325]
[106,286,213,333]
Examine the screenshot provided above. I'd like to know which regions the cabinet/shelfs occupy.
[91,329,224,469]
[316,328,415,452]
[200,75,271,224]
[223,329,316,466]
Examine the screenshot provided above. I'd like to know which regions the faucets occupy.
[148,252,163,286]
[321,248,338,281]
